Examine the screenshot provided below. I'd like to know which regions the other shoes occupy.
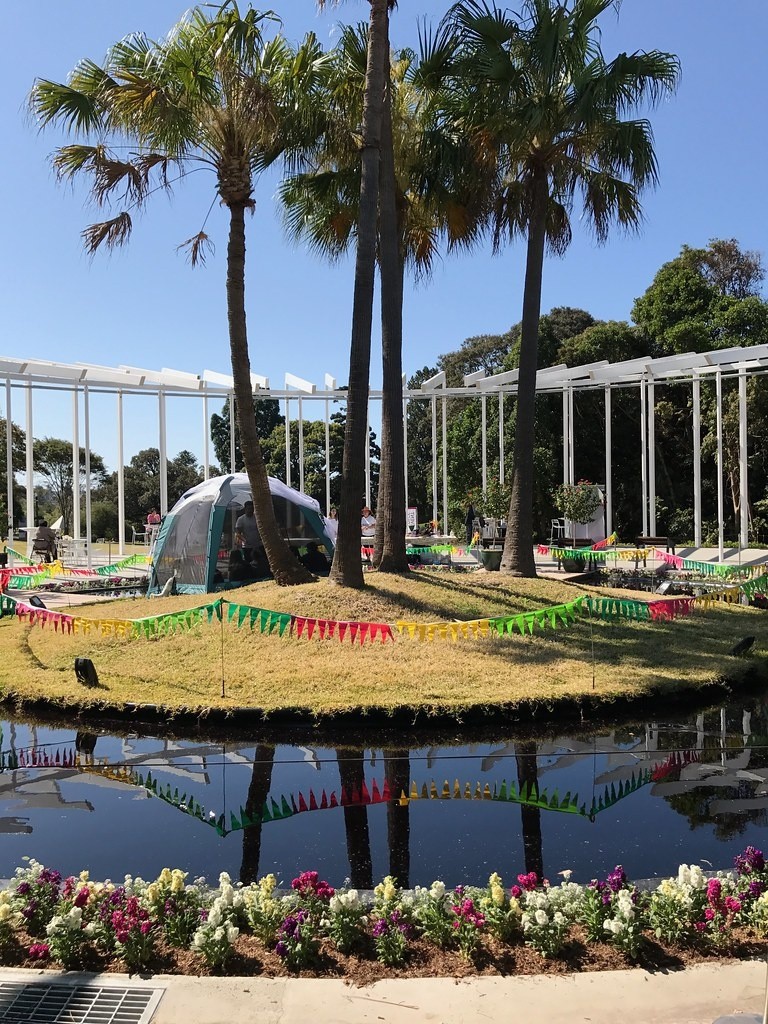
[362,560,370,562]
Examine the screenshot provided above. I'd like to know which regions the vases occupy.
[480,549,503,571]
[561,557,587,572]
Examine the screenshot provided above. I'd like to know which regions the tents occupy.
[148,473,335,599]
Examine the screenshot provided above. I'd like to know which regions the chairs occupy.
[29,538,107,566]
[132,526,159,546]
[550,519,570,548]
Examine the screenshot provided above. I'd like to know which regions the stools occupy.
[635,537,678,571]
[557,538,597,571]
[481,538,505,550]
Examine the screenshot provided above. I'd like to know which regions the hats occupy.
[361,507,370,510]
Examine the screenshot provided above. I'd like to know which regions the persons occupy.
[327,506,341,534]
[146,507,161,532]
[34,520,57,563]
[227,500,329,582]
[356,506,378,563]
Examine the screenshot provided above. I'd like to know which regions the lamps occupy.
[655,580,673,594]
[30,595,46,610]
[75,658,98,685]
[730,636,755,654]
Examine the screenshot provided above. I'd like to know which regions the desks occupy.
[60,539,87,566]
[335,536,457,565]
[143,525,161,534]
